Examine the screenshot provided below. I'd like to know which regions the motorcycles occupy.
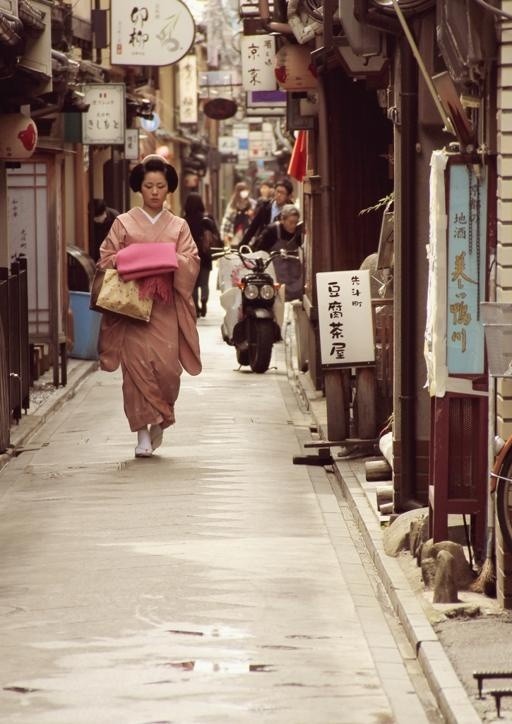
[209,243,304,377]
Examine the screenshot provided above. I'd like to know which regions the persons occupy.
[88,199,120,260]
[221,177,305,302]
[179,192,225,316]
[89,154,202,458]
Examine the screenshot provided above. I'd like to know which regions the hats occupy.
[281,203,300,219]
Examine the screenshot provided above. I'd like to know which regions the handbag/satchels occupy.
[89,266,153,324]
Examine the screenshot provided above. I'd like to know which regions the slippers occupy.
[147,425,166,449]
[134,444,154,459]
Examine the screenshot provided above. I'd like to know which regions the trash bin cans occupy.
[69,291,102,360]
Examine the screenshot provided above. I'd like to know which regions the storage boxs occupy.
[67,289,105,361]
